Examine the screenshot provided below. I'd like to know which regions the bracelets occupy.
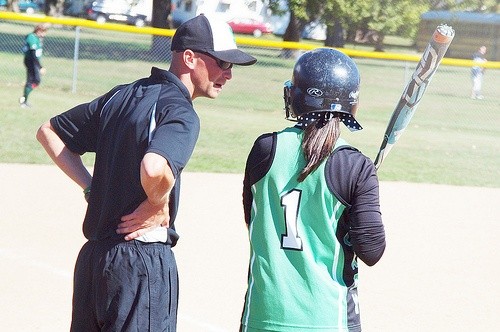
[83,186,92,194]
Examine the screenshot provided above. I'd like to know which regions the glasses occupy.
[176,49,233,69]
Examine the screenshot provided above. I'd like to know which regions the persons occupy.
[239,46,386,332]
[35,12,257,332]
[19,25,47,109]
[470,44,487,100]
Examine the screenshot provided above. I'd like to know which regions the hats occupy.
[170,13,257,66]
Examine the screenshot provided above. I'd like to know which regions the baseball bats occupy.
[374,24,455,170]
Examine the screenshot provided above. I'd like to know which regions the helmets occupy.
[290,47,361,121]
